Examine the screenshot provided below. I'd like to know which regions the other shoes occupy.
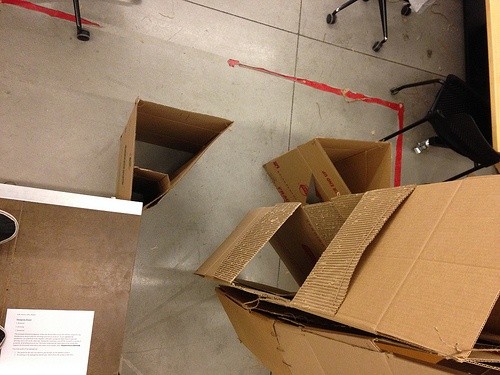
[0,210,19,243]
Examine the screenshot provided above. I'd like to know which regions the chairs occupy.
[378,73,500,182]
